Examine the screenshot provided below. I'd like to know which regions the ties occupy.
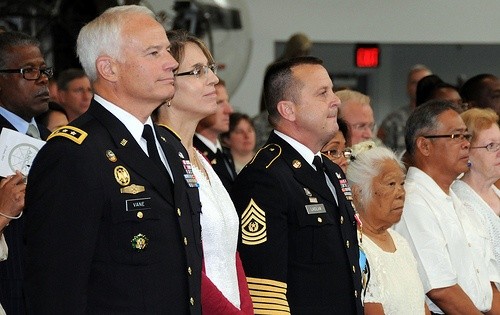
[312,156,326,184]
[27,124,40,139]
[142,124,172,183]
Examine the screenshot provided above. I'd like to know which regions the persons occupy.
[0,6,499,315]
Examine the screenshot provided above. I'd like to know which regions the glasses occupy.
[174,64,217,79]
[351,122,377,131]
[472,142,500,152]
[322,147,352,158]
[0,65,54,81]
[424,132,472,142]
